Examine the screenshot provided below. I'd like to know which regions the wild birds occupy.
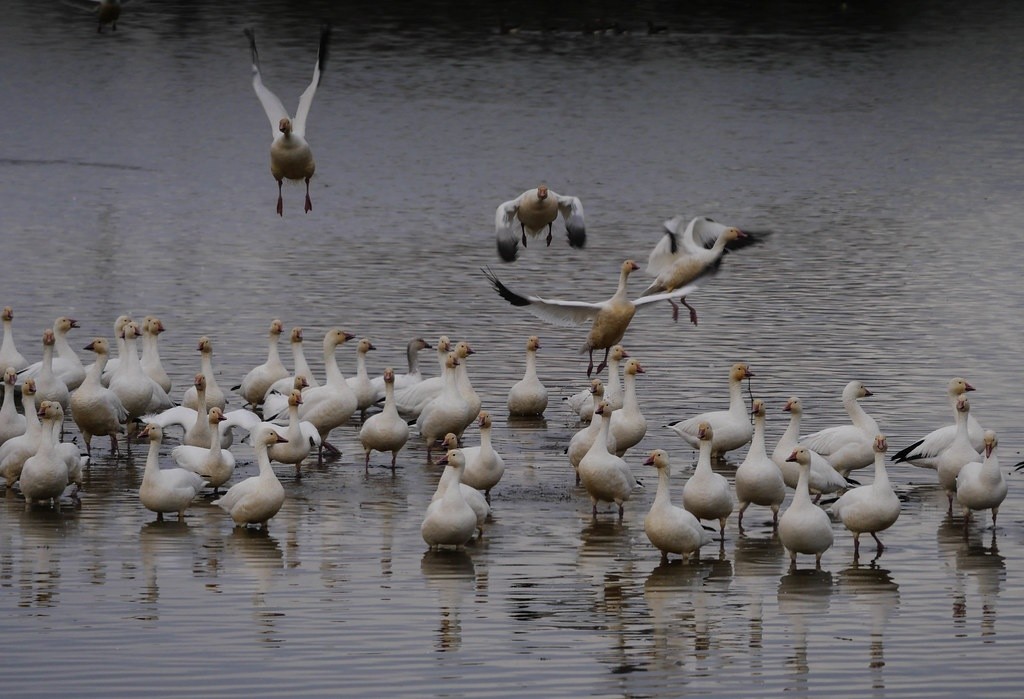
[495,186,586,263]
[482,260,699,379]
[1,304,1010,569]
[639,215,775,327]
[243,20,330,217]
